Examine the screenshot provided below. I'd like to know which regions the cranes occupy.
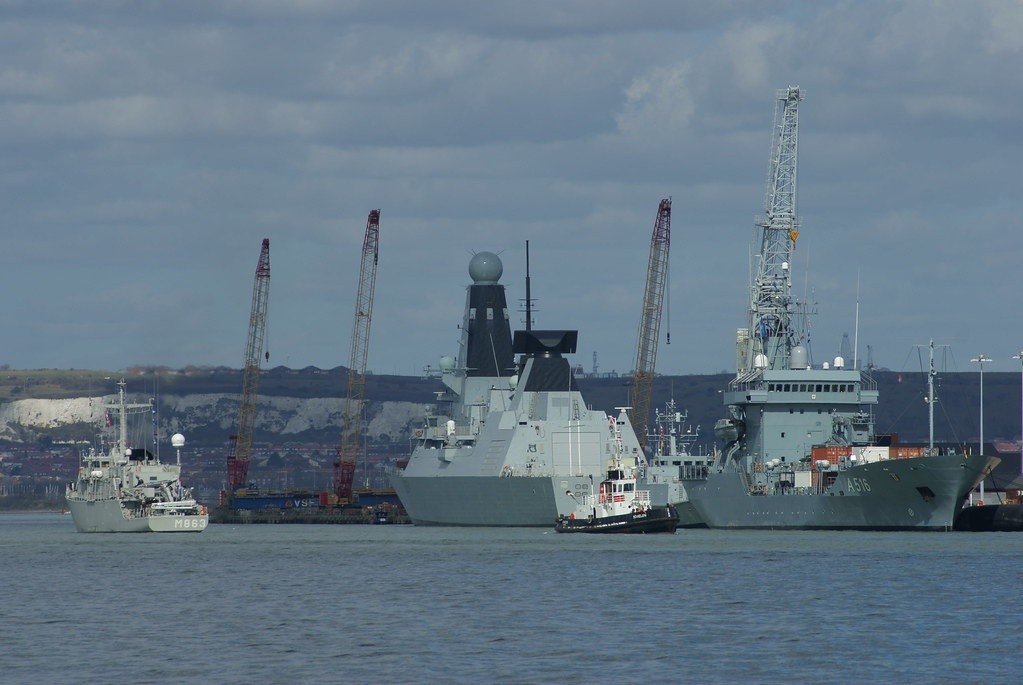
[316,206,383,507]
[226,238,270,492]
[628,197,673,452]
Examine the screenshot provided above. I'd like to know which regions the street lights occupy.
[970,355,992,506]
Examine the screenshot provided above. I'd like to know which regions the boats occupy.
[553,411,681,534]
[380,83,1004,532]
[62,378,211,534]
